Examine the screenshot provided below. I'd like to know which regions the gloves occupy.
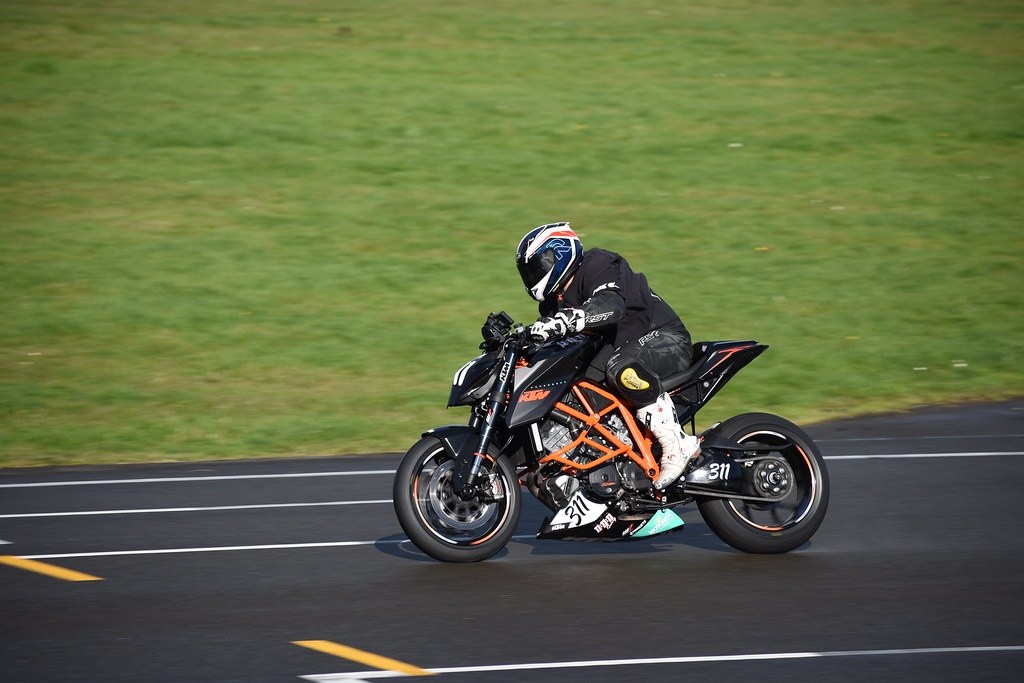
[529,306,586,344]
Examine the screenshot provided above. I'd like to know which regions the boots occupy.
[635,392,701,490]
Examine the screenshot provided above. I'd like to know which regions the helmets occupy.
[514,221,583,302]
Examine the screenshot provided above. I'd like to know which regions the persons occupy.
[516,221,701,489]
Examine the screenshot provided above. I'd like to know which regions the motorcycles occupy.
[393,309,831,565]
